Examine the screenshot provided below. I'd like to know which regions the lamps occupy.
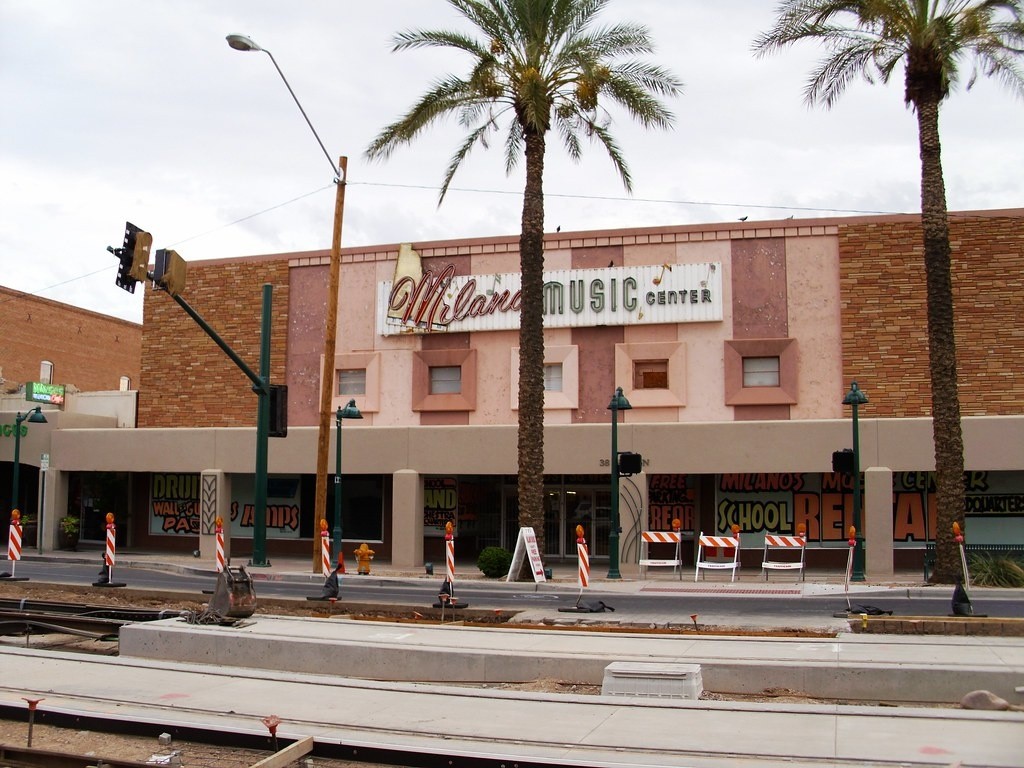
[653,263,671,285]
[701,263,716,287]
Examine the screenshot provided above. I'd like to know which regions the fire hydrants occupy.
[353,540,375,574]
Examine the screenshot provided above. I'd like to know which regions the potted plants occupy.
[21,513,38,546]
[58,515,81,551]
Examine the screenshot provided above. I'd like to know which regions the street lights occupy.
[227,32,351,575]
[328,399,363,578]
[12,405,49,519]
[832,380,871,582]
[607,386,642,580]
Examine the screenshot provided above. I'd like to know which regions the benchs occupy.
[924,544,1024,580]
